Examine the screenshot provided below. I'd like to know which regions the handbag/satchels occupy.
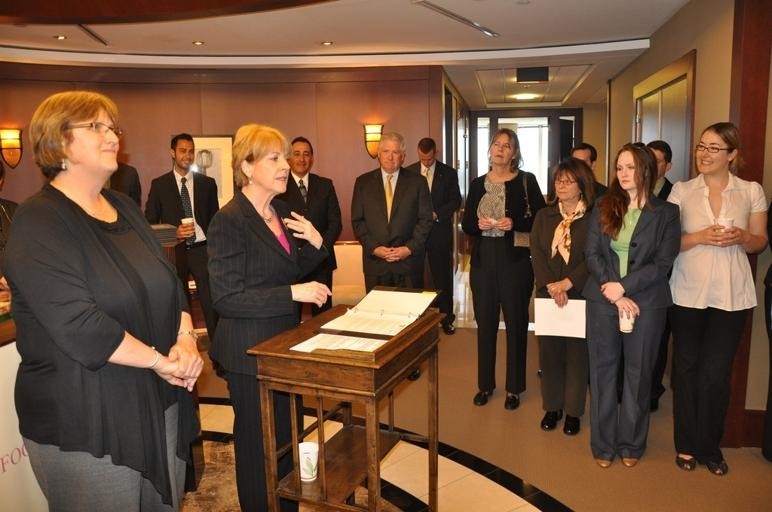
[514,230,531,247]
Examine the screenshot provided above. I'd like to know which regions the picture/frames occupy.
[167,131,236,212]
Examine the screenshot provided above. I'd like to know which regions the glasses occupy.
[696,145,729,153]
[554,178,576,185]
[623,142,649,155]
[64,121,124,138]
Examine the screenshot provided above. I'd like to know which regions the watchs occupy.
[175,330,200,342]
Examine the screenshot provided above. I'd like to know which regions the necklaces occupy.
[259,205,276,224]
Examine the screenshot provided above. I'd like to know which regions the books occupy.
[311,286,442,341]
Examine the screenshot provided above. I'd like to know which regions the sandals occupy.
[676,453,696,471]
[708,459,727,476]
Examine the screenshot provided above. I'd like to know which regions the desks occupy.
[243,302,448,512]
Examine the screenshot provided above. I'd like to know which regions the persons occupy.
[406,137,462,333]
[144,132,230,383]
[530,160,596,435]
[666,121,768,477]
[204,123,332,511]
[584,142,682,469]
[0,161,18,315]
[352,132,433,380]
[571,144,607,196]
[3,90,204,512]
[647,139,675,412]
[282,137,341,323]
[107,162,140,207]
[462,127,546,410]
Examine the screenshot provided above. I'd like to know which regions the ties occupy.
[181,177,196,245]
[422,168,431,192]
[299,179,308,204]
[384,175,393,220]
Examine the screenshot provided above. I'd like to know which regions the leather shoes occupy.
[443,324,455,335]
[474,390,492,405]
[563,415,579,434]
[541,411,563,430]
[409,370,419,380]
[505,391,520,409]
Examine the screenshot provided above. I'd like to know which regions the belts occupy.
[175,240,207,250]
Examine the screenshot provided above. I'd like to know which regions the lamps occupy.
[1,126,24,173]
[360,118,386,162]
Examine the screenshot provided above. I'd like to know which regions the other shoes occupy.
[650,399,658,411]
[622,457,637,467]
[598,459,611,467]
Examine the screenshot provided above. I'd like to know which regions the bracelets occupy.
[147,346,162,370]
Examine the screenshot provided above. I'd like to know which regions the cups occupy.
[181,218,195,229]
[297,442,319,481]
[713,218,735,245]
[619,308,636,334]
